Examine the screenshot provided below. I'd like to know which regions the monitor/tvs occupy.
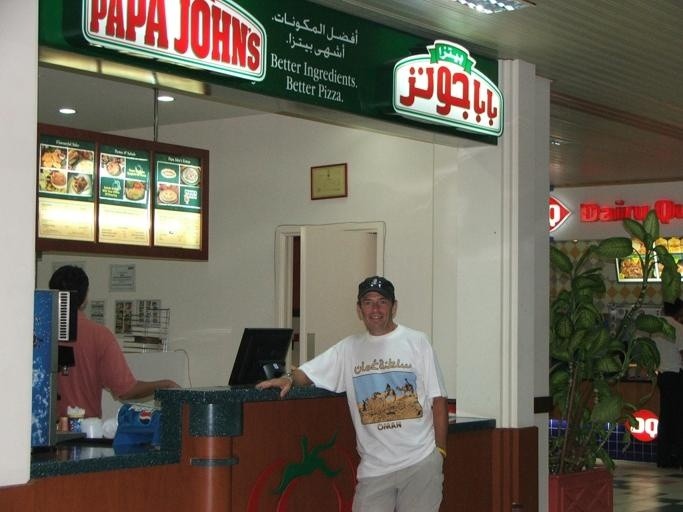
[228,328,294,387]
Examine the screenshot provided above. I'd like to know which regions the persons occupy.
[254,276,449,512]
[48,266,182,425]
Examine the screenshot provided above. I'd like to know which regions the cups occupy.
[55,414,83,435]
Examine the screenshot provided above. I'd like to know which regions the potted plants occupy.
[548,210,683,512]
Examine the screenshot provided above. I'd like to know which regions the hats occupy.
[49,265,89,296]
[358,277,394,301]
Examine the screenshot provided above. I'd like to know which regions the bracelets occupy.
[279,373,296,386]
[437,446,447,459]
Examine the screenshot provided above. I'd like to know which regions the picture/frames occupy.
[309,162,348,200]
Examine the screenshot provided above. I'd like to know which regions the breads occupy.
[160,189,177,202]
[50,171,67,185]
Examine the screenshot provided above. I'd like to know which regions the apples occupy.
[127,181,133,188]
[134,182,141,189]
